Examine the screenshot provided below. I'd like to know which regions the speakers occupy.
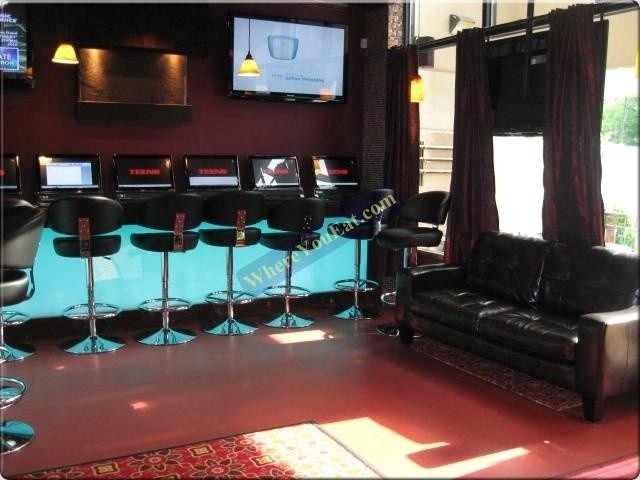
[415,37,434,67]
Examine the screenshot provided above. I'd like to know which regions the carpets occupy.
[0,416,388,478]
[411,332,587,413]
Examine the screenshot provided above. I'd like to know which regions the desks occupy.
[4,190,373,317]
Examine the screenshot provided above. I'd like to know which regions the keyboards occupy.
[35,191,104,202]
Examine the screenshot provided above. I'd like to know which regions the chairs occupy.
[374,189,455,337]
[260,194,326,329]
[1,195,46,457]
[44,193,123,355]
[199,187,268,337]
[329,185,398,321]
[130,190,201,345]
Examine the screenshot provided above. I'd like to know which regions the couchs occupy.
[401,229,640,422]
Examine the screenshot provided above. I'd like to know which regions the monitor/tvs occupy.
[487,19,610,136]
[0,0,36,81]
[227,11,349,106]
[0,155,24,197]
[312,155,360,190]
[184,154,242,194]
[33,151,103,192]
[251,154,302,193]
[111,153,176,190]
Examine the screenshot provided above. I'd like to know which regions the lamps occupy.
[47,4,81,66]
[234,4,262,78]
[410,0,427,103]
[449,14,476,37]
